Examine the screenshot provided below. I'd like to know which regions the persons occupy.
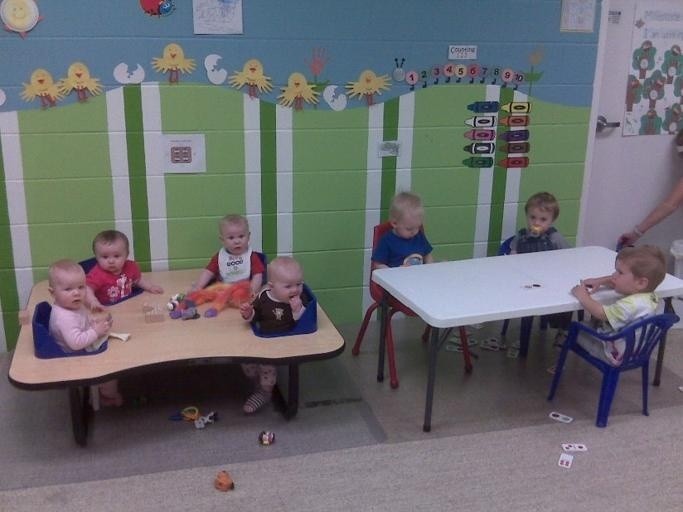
[509,193,572,348]
[617,173,683,250]
[570,246,667,367]
[369,193,434,269]
[236,256,306,414]
[46,260,123,409]
[86,231,165,314]
[189,213,265,299]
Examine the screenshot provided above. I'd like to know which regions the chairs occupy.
[78,257,144,306]
[547,313,679,427]
[353,221,472,388]
[32,302,107,359]
[250,283,317,339]
[249,251,268,285]
[498,235,578,356]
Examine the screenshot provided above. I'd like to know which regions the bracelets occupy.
[631,225,646,240]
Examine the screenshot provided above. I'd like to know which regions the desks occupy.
[9,269,346,445]
[371,245,683,432]
